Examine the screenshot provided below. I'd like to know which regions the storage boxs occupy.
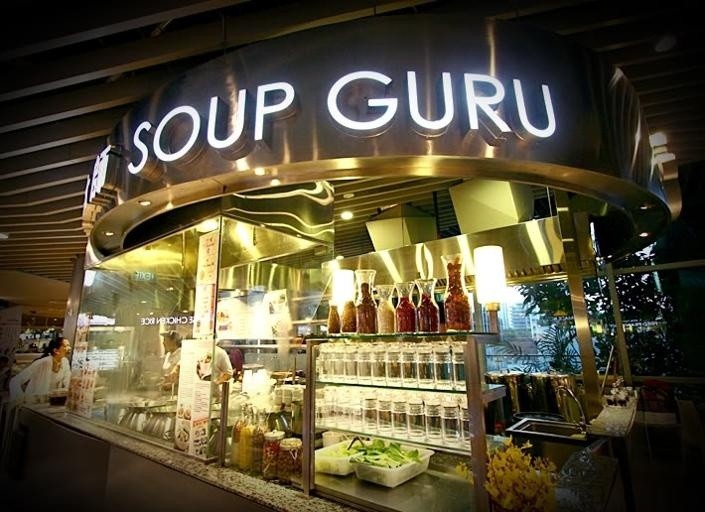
[314,438,374,475]
[350,444,436,489]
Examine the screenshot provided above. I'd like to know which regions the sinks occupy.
[505,418,580,438]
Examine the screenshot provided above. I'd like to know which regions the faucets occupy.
[555,385,586,429]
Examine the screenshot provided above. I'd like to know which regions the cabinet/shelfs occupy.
[302,331,506,509]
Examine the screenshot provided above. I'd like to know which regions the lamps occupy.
[473,242,508,336]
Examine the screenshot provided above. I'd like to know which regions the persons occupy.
[2,330,63,360]
[4,336,75,474]
[161,326,234,398]
[159,330,183,377]
[226,345,248,373]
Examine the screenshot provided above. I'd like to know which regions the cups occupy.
[270,383,305,412]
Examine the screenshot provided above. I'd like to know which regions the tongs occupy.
[345,436,369,455]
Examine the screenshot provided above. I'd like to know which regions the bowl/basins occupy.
[48,395,67,405]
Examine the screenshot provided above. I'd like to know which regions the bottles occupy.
[229,402,269,475]
[318,339,469,392]
[326,253,474,334]
[261,430,303,485]
[313,386,470,448]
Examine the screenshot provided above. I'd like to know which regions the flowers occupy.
[455,431,560,512]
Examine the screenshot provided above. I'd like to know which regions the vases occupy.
[322,249,475,336]
